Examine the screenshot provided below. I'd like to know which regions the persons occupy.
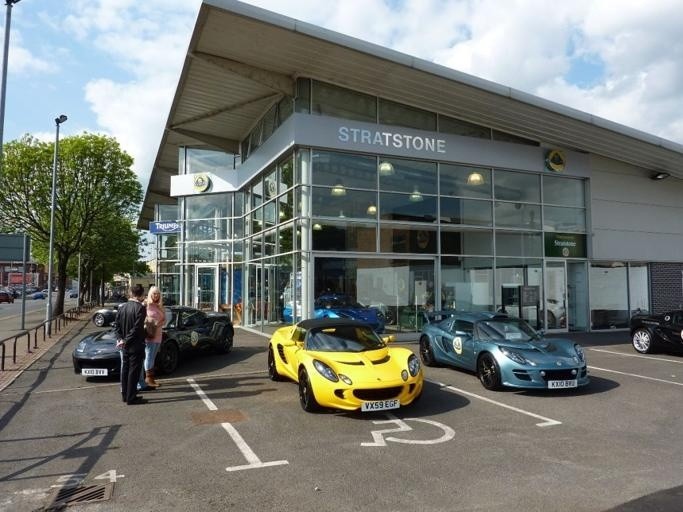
[113,283,167,405]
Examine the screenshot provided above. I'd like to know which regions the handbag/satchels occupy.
[142,318,156,339]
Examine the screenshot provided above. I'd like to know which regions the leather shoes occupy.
[127,399,147,405]
[121,390,140,395]
[139,386,156,391]
[122,396,142,402]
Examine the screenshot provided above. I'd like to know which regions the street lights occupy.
[0,0,20,156]
[44,115,69,337]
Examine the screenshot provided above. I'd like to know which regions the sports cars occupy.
[266,317,426,415]
[284,294,388,334]
[629,310,682,359]
[497,297,566,329]
[93,303,122,328]
[71,305,235,380]
[418,310,590,392]
[376,301,457,333]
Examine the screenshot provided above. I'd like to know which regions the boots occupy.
[145,368,161,387]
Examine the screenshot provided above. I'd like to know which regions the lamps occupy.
[650,171,670,181]
[310,159,481,231]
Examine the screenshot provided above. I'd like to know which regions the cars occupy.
[0,281,79,303]
